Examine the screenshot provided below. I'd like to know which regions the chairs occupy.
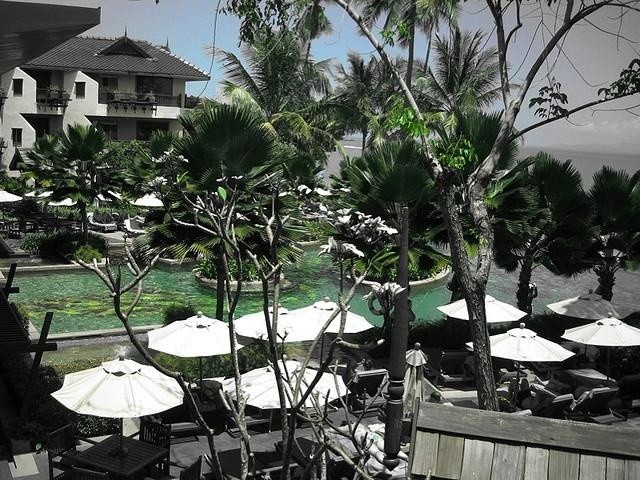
[346,348,639,423]
[156,376,274,435]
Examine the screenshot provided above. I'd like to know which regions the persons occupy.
[509,377,548,408]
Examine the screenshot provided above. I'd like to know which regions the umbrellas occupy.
[560,312,637,387]
[50,354,185,451]
[292,296,376,368]
[545,288,626,358]
[436,292,529,324]
[402,341,430,420]
[221,352,353,413]
[463,322,575,409]
[146,310,253,400]
[225,302,321,345]
